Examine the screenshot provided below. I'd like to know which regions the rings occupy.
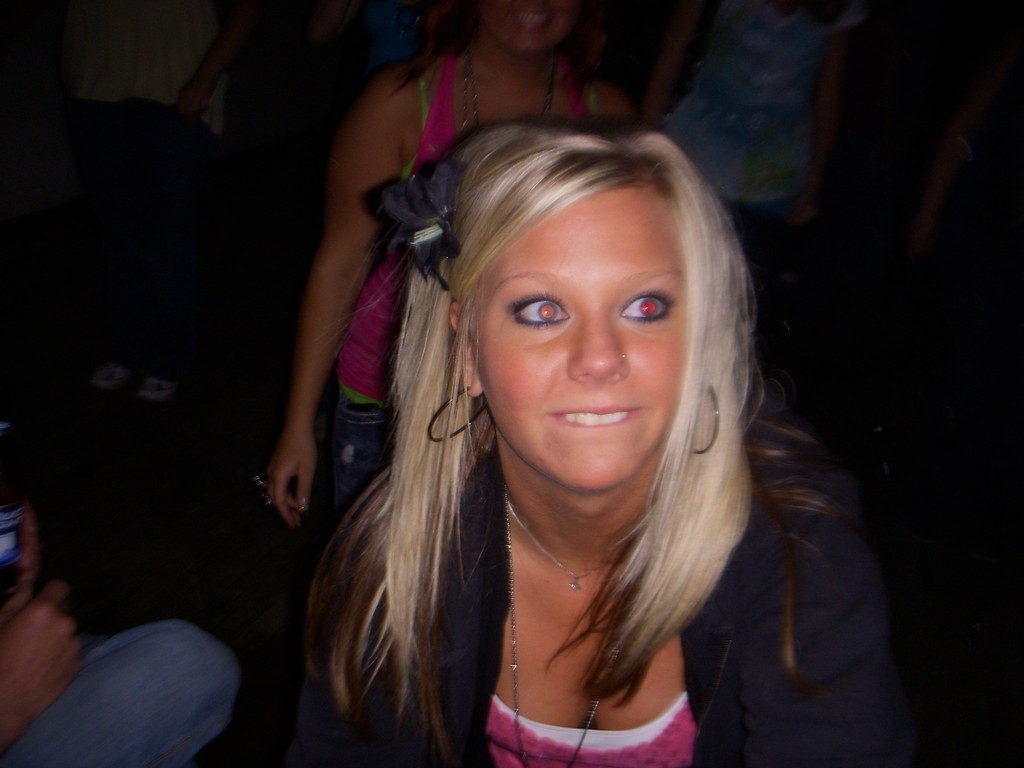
[261,493,273,506]
[254,476,268,490]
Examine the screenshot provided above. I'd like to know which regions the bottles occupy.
[0,417,27,607]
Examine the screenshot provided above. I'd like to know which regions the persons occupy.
[641,0,849,333]
[52,0,264,423]
[253,0,640,531]
[0,495,241,768]
[906,32,1024,534]
[287,118,921,768]
[303,0,419,83]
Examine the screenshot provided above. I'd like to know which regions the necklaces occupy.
[501,485,621,768]
[456,45,481,138]
